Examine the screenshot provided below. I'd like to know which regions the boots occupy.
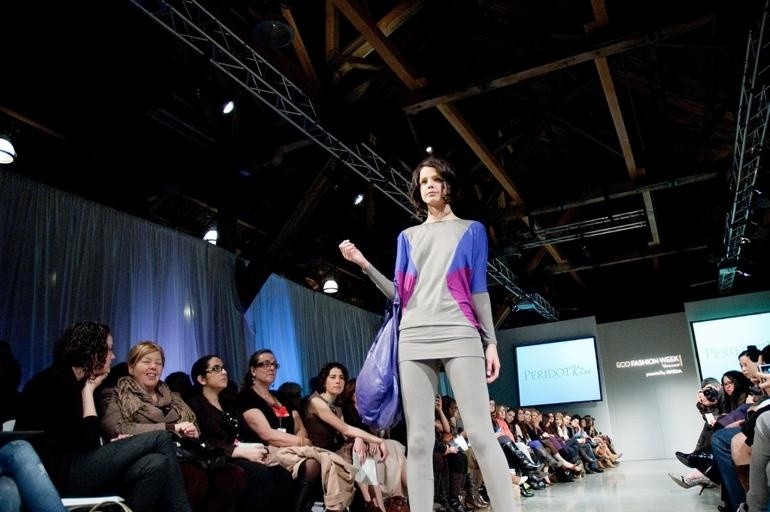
[441,475,455,512]
[451,469,488,511]
[434,473,449,512]
[502,437,623,497]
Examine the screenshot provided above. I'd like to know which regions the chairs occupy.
[60,490,133,512]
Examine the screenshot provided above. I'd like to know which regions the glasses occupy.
[204,366,228,373]
[255,361,281,370]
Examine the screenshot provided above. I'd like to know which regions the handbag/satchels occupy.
[355,283,401,429]
[169,430,225,473]
[387,494,410,511]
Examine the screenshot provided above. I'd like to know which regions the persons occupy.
[665,342,770,512]
[95,338,249,511]
[188,353,325,511]
[21,319,193,511]
[488,399,623,496]
[335,153,520,511]
[302,361,491,511]
[0,337,68,511]
[238,348,357,512]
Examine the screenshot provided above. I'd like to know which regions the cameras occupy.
[760,364,770,374]
[704,388,718,401]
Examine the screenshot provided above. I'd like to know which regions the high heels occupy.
[675,448,717,475]
[669,472,710,495]
